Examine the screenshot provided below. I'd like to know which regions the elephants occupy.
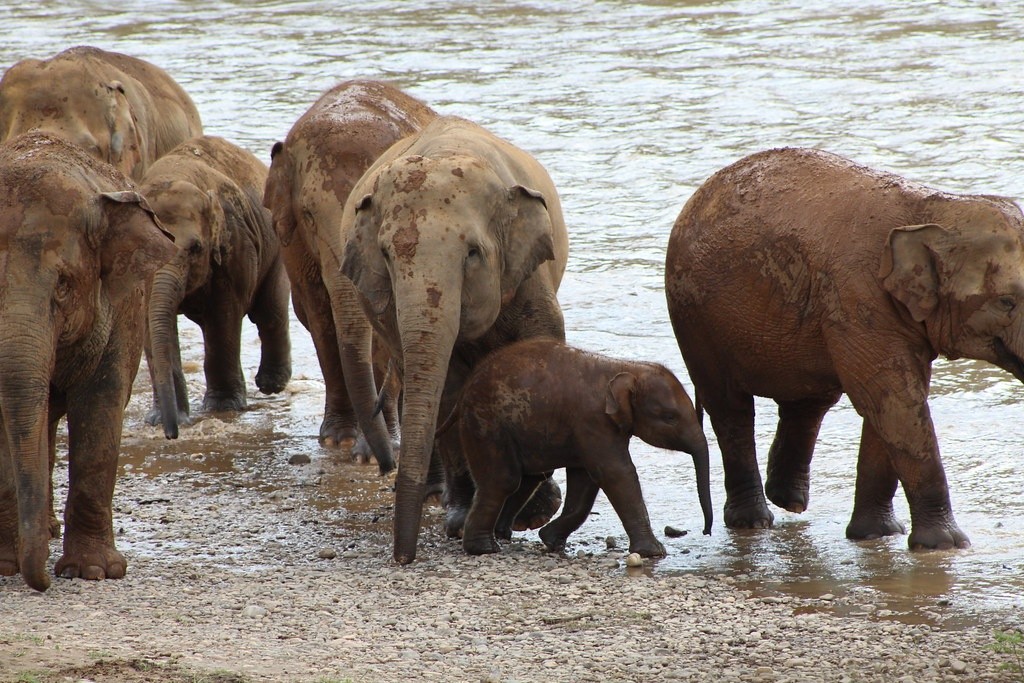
[0,45,1024,594]
